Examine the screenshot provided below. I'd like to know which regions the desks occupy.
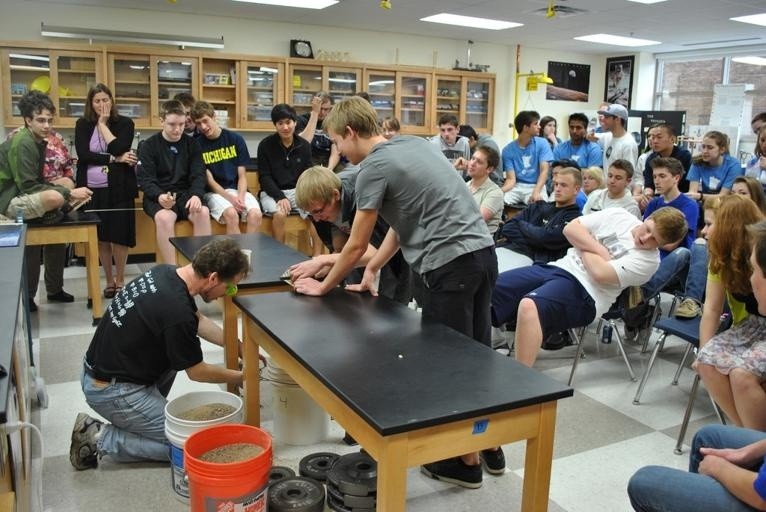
[24,212,104,327]
[232,289,573,512]
[168,231,314,396]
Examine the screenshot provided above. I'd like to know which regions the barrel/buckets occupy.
[183,423,275,512]
[162,390,244,505]
[260,356,333,447]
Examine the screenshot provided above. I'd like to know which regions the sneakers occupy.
[673,297,701,317]
[34,206,65,226]
[624,326,638,341]
[479,446,507,475]
[420,456,484,490]
[637,328,652,345]
[68,410,103,471]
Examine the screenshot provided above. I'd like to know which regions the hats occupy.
[597,103,629,121]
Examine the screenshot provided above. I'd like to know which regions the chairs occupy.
[568,296,727,456]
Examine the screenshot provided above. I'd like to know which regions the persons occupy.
[7,124,74,311]
[295,91,341,171]
[290,94,505,491]
[137,97,211,269]
[68,235,268,471]
[74,82,137,299]
[256,102,324,258]
[625,218,766,512]
[0,93,94,225]
[173,92,201,139]
[189,99,263,234]
[294,162,412,307]
[381,98,765,369]
[691,192,766,433]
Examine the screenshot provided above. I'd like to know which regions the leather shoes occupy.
[542,328,566,350]
[114,285,122,295]
[46,290,75,303]
[21,296,38,312]
[103,286,115,298]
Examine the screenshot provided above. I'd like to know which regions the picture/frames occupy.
[604,55,634,111]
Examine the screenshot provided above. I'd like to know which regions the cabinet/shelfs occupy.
[108,52,198,129]
[200,58,285,130]
[363,69,432,138]
[288,63,363,116]
[0,47,106,128]
[431,74,494,137]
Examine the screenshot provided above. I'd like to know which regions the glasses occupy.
[303,202,327,215]
[227,281,238,298]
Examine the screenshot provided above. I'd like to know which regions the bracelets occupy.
[111,155,117,163]
